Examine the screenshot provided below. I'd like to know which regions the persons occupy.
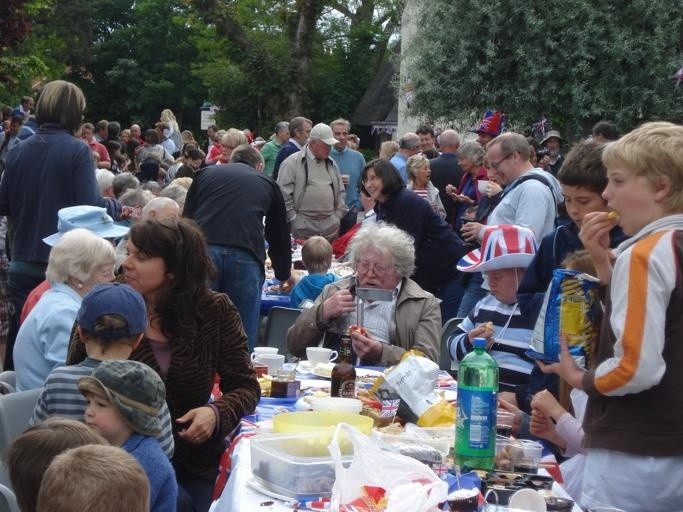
[37,445,151,512]
[447,141,495,236]
[529,388,590,465]
[343,126,441,187]
[65,214,260,512]
[403,154,448,227]
[20,206,130,325]
[428,130,467,231]
[182,145,295,352]
[591,122,623,145]
[532,129,567,180]
[460,133,564,253]
[29,282,174,458]
[13,229,116,392]
[535,122,683,511]
[4,415,110,512]
[123,108,208,179]
[258,117,313,180]
[0,81,122,372]
[468,110,507,146]
[89,170,193,227]
[275,124,349,244]
[331,119,367,236]
[447,226,539,413]
[516,140,632,401]
[78,119,124,173]
[0,96,37,151]
[289,236,344,311]
[77,359,177,512]
[206,126,266,165]
[287,221,442,366]
[358,160,470,327]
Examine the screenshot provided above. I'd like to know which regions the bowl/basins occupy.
[272,411,374,456]
[546,497,575,512]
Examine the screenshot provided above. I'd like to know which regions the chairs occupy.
[265,306,325,347]
[0,371,17,394]
[0,484,20,512]
[441,318,464,372]
[0,390,43,484]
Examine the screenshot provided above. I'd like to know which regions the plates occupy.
[310,366,333,378]
[354,368,384,384]
[435,390,457,402]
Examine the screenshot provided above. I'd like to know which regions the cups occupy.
[509,442,543,474]
[510,489,546,512]
[446,490,479,512]
[254,354,285,373]
[478,180,490,194]
[310,398,362,411]
[251,347,279,363]
[306,347,338,363]
[497,412,513,438]
[495,438,512,472]
[461,216,482,247]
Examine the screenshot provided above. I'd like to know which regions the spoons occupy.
[455,466,461,491]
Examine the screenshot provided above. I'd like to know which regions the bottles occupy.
[332,335,356,397]
[456,337,499,470]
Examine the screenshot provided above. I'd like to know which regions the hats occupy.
[540,131,562,144]
[467,110,502,136]
[74,357,168,438]
[73,281,148,343]
[42,204,132,248]
[454,224,538,274]
[308,123,340,146]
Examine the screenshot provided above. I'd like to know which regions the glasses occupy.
[484,149,521,171]
[157,216,185,257]
[355,262,395,278]
